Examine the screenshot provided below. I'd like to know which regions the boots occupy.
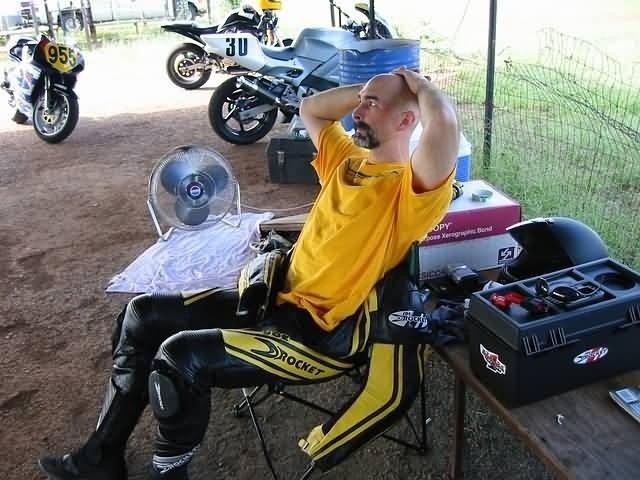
[37,376,148,479]
[152,419,202,479]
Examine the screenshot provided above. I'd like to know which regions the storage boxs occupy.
[418,180,521,282]
[266,138,317,185]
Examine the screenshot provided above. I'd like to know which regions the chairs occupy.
[232,240,427,480]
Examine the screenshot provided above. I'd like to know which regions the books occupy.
[607,384,640,423]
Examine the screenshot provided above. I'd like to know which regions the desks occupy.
[422,268,640,480]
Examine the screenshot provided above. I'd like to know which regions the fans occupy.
[147,143,241,239]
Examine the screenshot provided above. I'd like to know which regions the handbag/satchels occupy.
[235,246,289,329]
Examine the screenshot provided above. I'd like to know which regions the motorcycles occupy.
[200,3,397,144]
[160,0,294,89]
[0,29,85,144]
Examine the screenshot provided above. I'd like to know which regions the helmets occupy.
[493,212,610,285]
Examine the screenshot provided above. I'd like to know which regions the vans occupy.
[20,0,206,32]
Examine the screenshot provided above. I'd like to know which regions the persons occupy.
[34,63,461,480]
